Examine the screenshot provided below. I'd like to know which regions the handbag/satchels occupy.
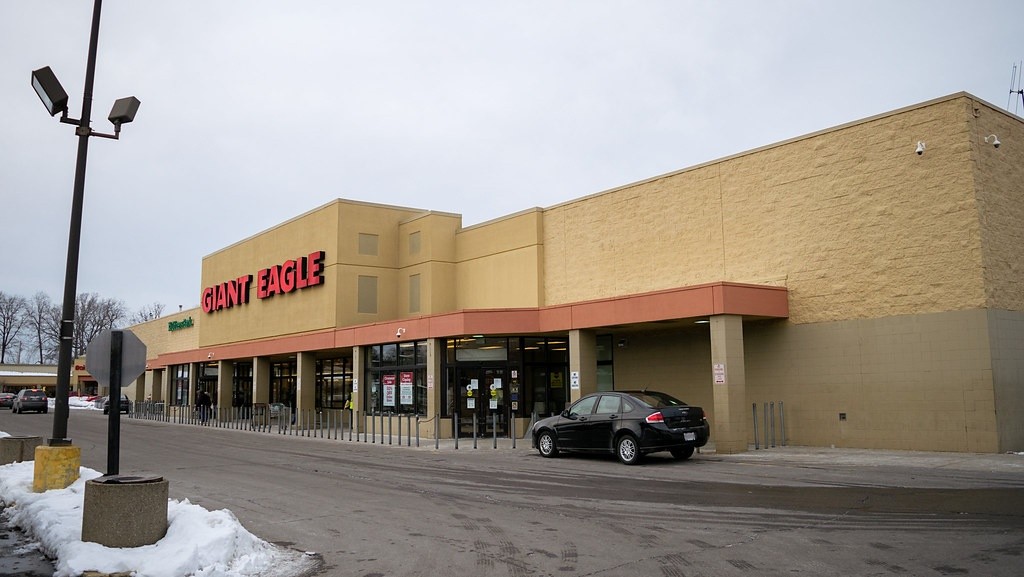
[210,403,213,409]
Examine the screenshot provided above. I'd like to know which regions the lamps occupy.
[207,352,214,359]
[86,94,142,142]
[396,327,406,338]
[146,363,151,368]
[984,134,1001,149]
[30,64,77,127]
[914,141,925,155]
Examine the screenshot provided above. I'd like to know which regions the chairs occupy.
[604,400,617,412]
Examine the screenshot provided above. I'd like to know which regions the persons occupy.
[344,384,353,429]
[147,394,151,403]
[197,391,211,419]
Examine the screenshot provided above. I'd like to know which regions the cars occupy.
[533,383,710,466]
[13,389,48,415]
[0,393,15,409]
[104,394,133,415]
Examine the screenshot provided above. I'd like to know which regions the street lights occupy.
[32,0,139,492]
